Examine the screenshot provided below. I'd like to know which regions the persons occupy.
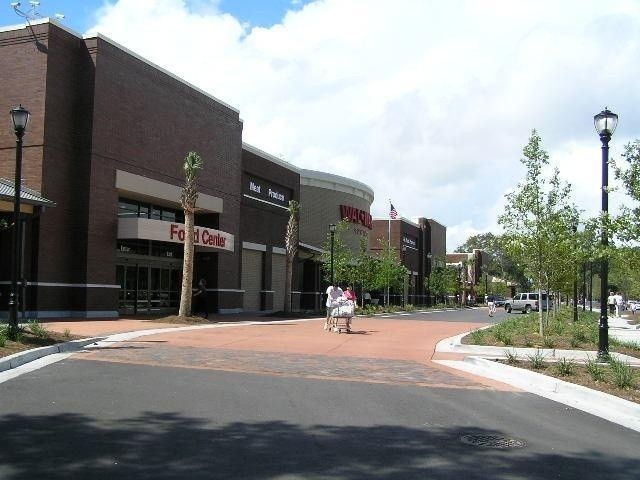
[343,283,358,331]
[324,283,348,332]
[193,278,208,318]
[467,292,495,317]
[608,292,637,318]
[364,291,371,305]
[577,299,584,305]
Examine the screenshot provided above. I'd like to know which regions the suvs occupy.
[485,294,508,308]
[457,294,478,307]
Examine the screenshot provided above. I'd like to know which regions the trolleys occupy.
[328,297,355,335]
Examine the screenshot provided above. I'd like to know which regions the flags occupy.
[390,204,398,219]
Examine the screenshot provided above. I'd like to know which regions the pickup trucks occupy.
[504,292,551,313]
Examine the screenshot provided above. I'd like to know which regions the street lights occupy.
[7,101,33,334]
[568,217,582,321]
[459,266,469,303]
[592,105,619,361]
[329,222,337,287]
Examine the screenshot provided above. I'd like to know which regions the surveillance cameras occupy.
[10,2,21,6]
[55,14,65,19]
[29,1,40,7]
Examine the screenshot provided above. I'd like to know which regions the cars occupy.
[627,300,640,313]
